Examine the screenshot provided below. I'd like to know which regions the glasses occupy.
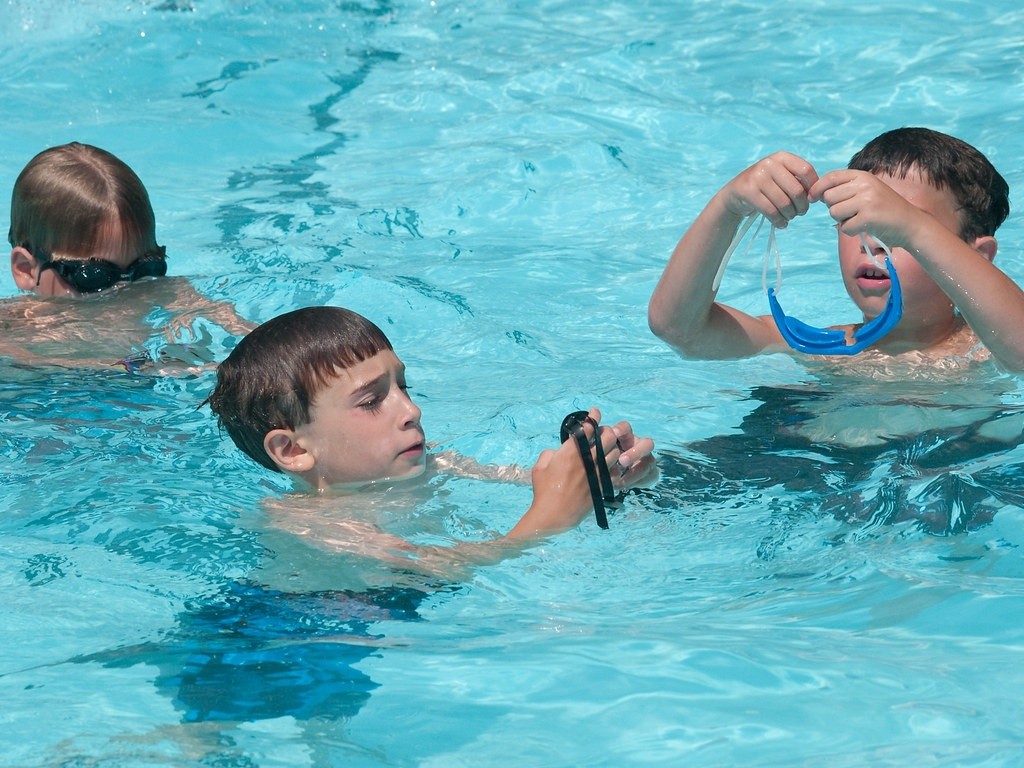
[561,411,641,530]
[22,245,167,292]
[712,211,903,356]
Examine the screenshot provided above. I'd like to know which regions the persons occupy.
[648,127,1024,376]
[195,304,661,538]
[7,141,168,299]
